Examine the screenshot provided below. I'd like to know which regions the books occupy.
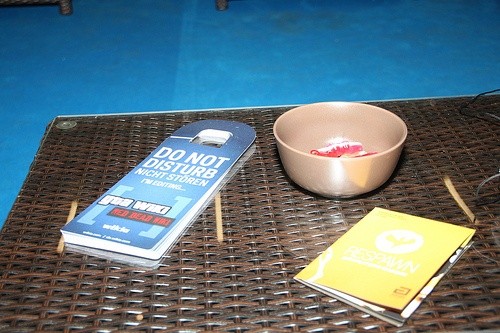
[291,206,476,329]
[60,119,258,271]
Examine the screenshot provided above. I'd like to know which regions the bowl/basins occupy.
[273,101,408,199]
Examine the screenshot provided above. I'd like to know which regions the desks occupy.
[0,91,500,332]
